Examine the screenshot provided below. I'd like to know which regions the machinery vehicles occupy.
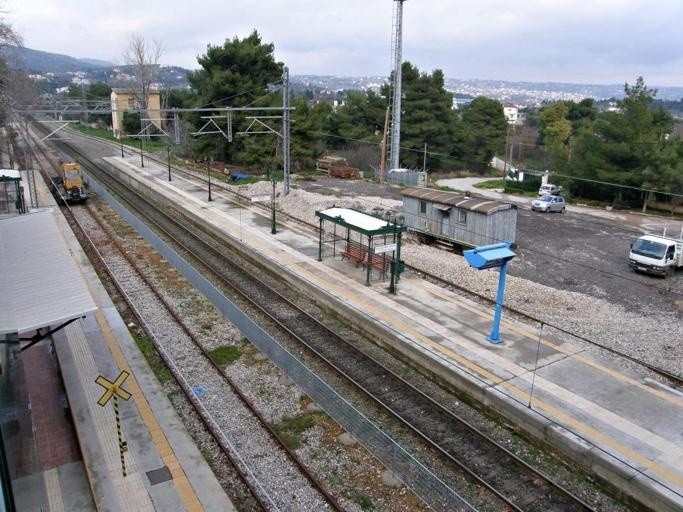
[51,161,89,207]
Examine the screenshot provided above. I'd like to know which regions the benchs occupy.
[339,238,392,282]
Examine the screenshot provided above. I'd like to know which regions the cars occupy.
[530,194,565,213]
[538,184,561,195]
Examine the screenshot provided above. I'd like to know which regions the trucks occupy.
[627,232,683,277]
